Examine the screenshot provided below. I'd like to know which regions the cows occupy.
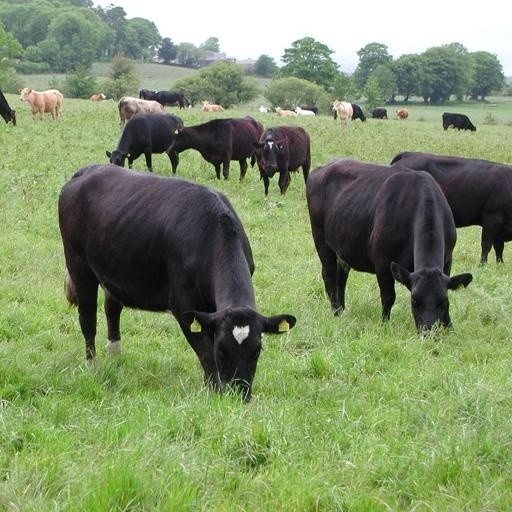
[306,159,472,336]
[441,111,476,133]
[0,89,16,127]
[250,125,312,196]
[390,151,511,266]
[171,115,264,181]
[330,97,409,126]
[259,104,317,118]
[106,112,184,175]
[202,100,223,112]
[57,163,297,404]
[90,93,106,102]
[118,90,185,128]
[19,89,63,122]
